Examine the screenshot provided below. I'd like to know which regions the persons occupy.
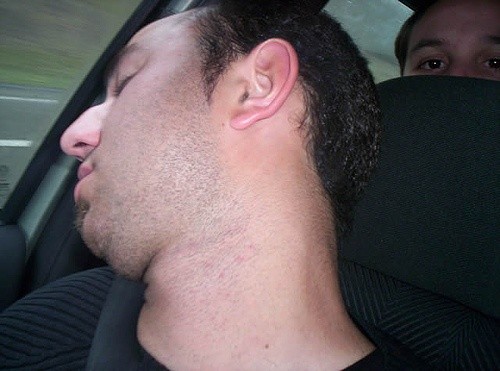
[394,0,500,80]
[60,0,427,371]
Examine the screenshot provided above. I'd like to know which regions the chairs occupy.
[1,75,500,371]
